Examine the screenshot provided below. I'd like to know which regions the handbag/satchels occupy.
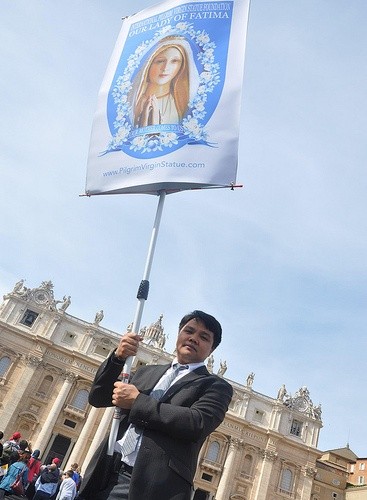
[10,470,25,496]
[41,471,58,484]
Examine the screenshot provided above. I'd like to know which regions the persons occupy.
[0,431,82,500]
[74,310,233,500]
[11,278,322,421]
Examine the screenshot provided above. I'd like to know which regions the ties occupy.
[122,363,189,456]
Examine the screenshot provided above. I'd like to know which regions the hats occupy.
[54,458,59,463]
[13,432,21,438]
[32,450,40,458]
